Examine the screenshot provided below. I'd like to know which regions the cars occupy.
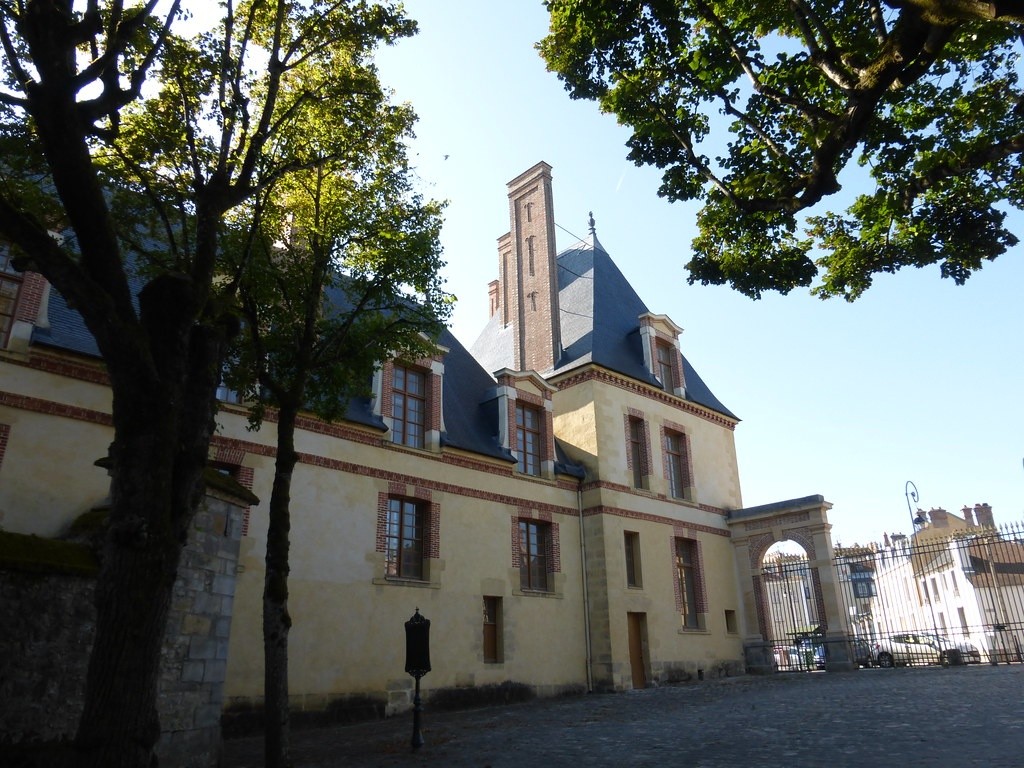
[873,631,981,668]
[800,637,874,668]
[773,645,806,671]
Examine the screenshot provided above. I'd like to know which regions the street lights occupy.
[904,480,948,670]
